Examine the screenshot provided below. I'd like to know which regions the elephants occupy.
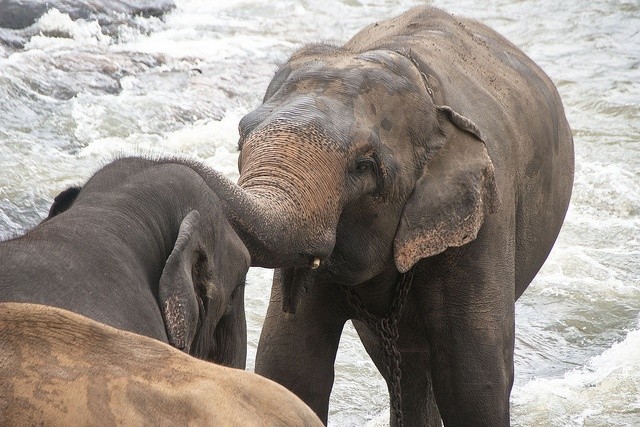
[176,5,575,427]
[1,157,328,427]
